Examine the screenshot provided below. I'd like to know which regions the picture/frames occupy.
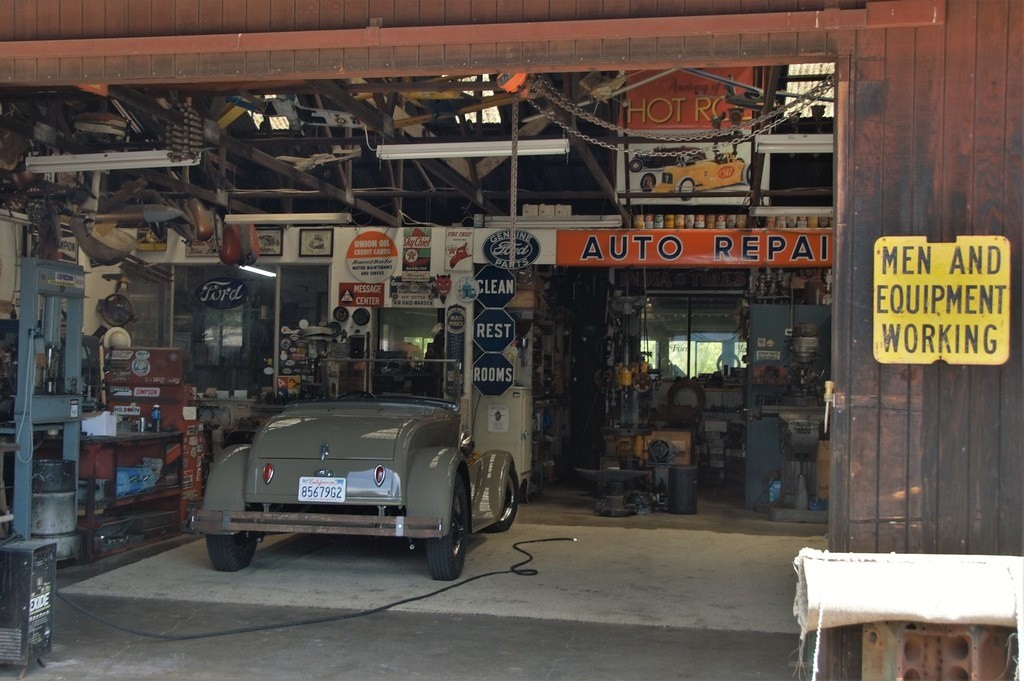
[299,228,334,258]
[255,228,284,256]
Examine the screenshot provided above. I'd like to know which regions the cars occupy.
[190,388,520,584]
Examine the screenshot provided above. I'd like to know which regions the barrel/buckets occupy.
[669,464,698,514]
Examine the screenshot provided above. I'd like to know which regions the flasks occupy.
[138,415,145,432]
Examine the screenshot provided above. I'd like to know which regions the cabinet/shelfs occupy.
[80,429,185,563]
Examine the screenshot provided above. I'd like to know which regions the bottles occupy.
[151,403,161,432]
[794,474,808,510]
[614,360,639,389]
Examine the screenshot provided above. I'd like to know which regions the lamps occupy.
[473,214,622,230]
[234,263,277,279]
[223,211,353,228]
[374,135,571,163]
[751,205,835,219]
[24,148,200,175]
[755,132,835,154]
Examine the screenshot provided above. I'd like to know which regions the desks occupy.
[704,388,743,412]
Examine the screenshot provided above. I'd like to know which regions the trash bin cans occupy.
[669,464,698,515]
[0,543,58,666]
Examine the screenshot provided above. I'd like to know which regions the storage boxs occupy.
[692,445,701,455]
[538,203,554,216]
[704,419,730,433]
[712,455,724,461]
[80,410,118,437]
[705,432,721,440]
[707,439,724,448]
[216,391,229,399]
[522,204,538,216]
[768,477,789,507]
[127,510,181,538]
[555,204,571,216]
[709,448,724,455]
[634,429,692,465]
[233,389,248,400]
[709,460,725,468]
[731,367,748,378]
